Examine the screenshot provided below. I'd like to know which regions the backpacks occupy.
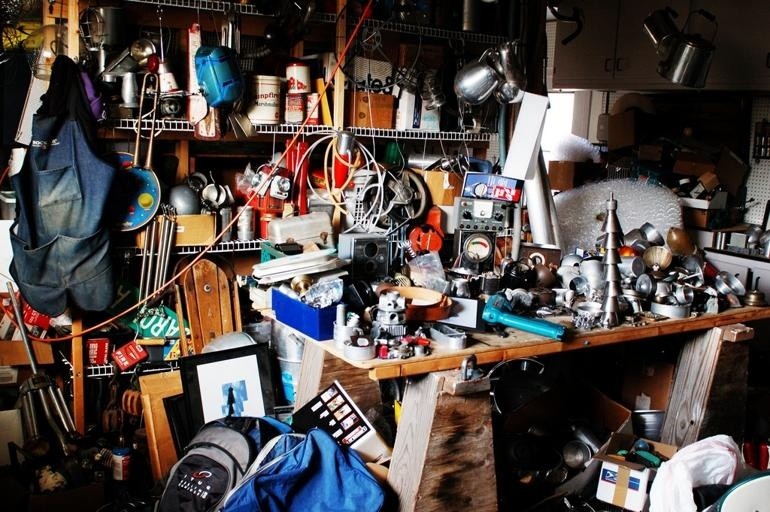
[146,413,294,512]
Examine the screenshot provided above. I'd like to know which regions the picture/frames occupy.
[178,343,275,437]
[137,370,195,485]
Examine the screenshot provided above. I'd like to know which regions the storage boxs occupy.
[331,90,395,129]
[592,433,679,511]
[136,212,216,249]
[493,384,634,511]
[608,108,657,151]
[549,161,602,191]
[0,292,56,365]
[409,167,464,206]
[622,362,674,411]
[673,139,748,197]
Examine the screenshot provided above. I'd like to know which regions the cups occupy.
[643,4,682,51]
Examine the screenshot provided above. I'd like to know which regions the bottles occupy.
[110,447,131,481]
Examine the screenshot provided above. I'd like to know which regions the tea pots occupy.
[654,8,719,90]
[452,47,507,108]
[492,38,529,107]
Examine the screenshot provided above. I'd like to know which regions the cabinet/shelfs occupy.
[0,0,512,438]
[552,0,770,91]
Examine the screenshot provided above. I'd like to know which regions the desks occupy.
[251,274,770,512]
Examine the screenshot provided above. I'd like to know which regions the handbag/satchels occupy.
[220,428,386,511]
